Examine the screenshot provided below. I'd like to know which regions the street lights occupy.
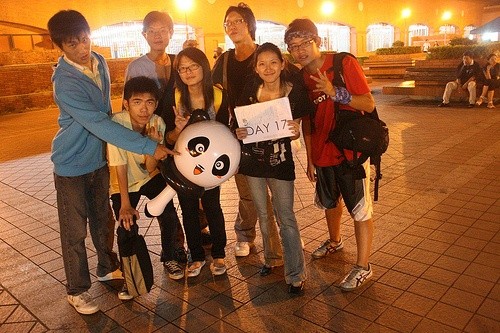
[177,0,191,40]
[443,11,452,45]
[402,8,410,46]
[322,2,334,52]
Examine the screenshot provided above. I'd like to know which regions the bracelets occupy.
[329,85,352,106]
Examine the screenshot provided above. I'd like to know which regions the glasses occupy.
[223,19,244,27]
[177,64,201,74]
[287,37,314,53]
[142,30,169,36]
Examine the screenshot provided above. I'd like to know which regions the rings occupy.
[123,221,126,224]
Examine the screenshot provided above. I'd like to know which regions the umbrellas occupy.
[469,16,500,41]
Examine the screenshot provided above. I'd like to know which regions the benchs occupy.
[382,60,500,107]
[364,60,413,79]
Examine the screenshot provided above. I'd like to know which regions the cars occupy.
[448,37,478,46]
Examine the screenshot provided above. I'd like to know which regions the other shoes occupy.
[235,242,250,257]
[467,103,476,108]
[474,96,485,108]
[288,281,305,295]
[188,260,205,277]
[301,238,305,249]
[487,101,495,109]
[438,102,449,107]
[259,263,272,276]
[174,247,188,265]
[213,261,226,276]
[201,229,212,248]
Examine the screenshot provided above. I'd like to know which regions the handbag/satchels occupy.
[116,215,154,297]
[331,109,389,165]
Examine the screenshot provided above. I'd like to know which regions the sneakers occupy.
[310,236,343,259]
[97,267,124,280]
[163,260,185,280]
[117,284,133,300]
[339,262,372,292]
[68,293,100,315]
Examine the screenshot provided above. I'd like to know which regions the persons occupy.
[476,54,500,108]
[284,19,374,288]
[422,39,431,53]
[235,42,304,295]
[47,9,181,315]
[106,10,231,299]
[437,52,480,108]
[433,41,439,47]
[212,2,305,257]
